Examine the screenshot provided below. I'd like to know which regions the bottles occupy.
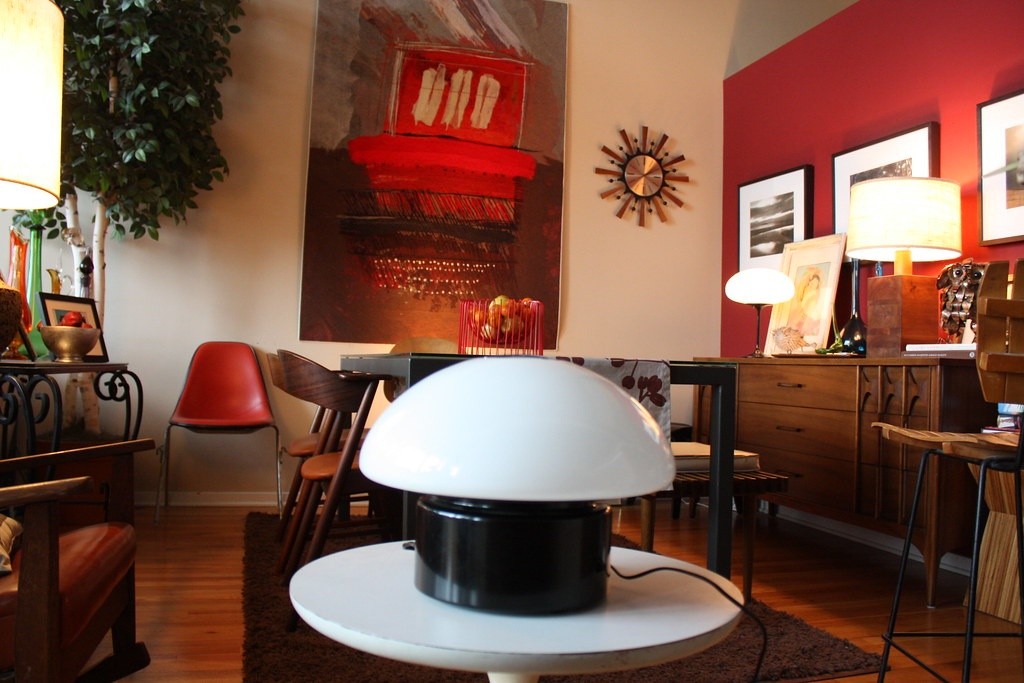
[2,225,33,359]
[17,209,48,358]
[838,258,866,355]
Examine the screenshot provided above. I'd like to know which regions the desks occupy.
[0,359,144,529]
[289,538,747,683]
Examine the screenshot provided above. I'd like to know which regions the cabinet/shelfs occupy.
[692,356,999,611]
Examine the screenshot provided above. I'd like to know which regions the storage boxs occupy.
[866,277,939,359]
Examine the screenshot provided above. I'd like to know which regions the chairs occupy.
[265,347,393,633]
[875,257,1024,683]
[0,438,156,683]
[161,341,283,519]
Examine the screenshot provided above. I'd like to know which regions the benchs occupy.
[621,472,790,606]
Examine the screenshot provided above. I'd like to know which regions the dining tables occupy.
[341,355,737,585]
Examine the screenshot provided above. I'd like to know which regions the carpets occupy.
[241,511,890,683]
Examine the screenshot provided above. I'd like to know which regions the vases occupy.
[2,225,34,361]
[17,224,48,361]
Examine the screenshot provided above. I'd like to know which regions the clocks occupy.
[596,124,690,228]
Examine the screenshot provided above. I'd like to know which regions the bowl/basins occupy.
[41,326,100,362]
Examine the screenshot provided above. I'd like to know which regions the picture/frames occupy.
[832,121,940,267]
[975,87,1024,247]
[39,291,109,364]
[738,164,815,275]
[763,233,848,357]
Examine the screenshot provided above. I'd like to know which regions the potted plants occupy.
[45,0,246,530]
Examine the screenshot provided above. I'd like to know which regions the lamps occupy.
[358,355,768,683]
[0,0,64,361]
[845,177,962,275]
[725,266,795,359]
